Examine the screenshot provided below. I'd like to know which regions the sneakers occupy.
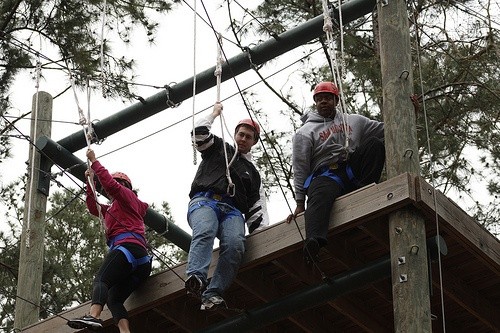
[67,313,103,330]
[200,296,228,311]
[185,275,202,298]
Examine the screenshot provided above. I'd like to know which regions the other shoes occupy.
[303,238,320,266]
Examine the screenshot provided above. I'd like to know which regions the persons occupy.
[67,148,152,333]
[184,101,270,311]
[286,81,421,274]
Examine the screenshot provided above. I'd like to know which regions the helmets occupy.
[111,172,132,190]
[313,82,339,106]
[235,119,260,144]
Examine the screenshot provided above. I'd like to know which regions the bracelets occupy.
[297,202,304,207]
